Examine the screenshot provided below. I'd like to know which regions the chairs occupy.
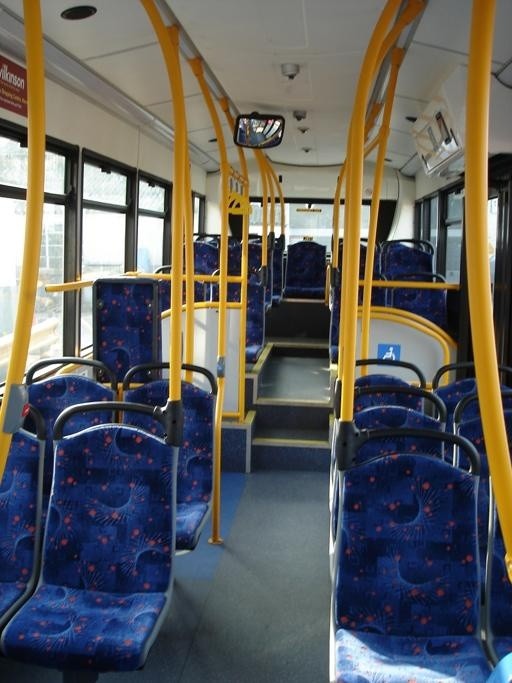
[151,229,328,380]
[330,239,512,683]
[2,275,218,683]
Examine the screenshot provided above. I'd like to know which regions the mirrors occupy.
[233,110,286,148]
